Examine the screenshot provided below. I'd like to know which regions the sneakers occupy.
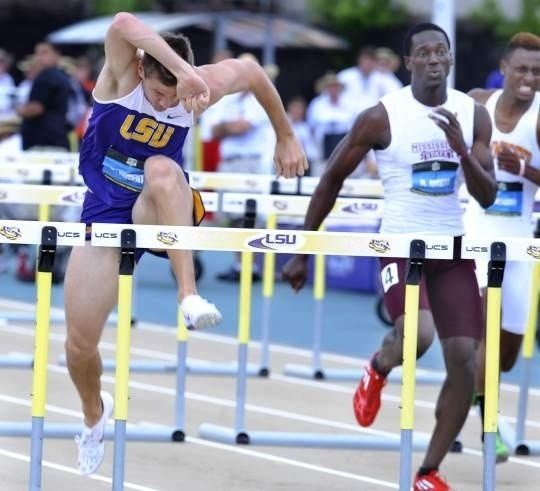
[352,350,391,427]
[412,469,454,491]
[181,293,221,330]
[480,429,510,463]
[75,389,114,476]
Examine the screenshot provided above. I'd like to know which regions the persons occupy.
[467,27,540,462]
[61,10,310,474]
[275,19,499,490]
[1,37,484,292]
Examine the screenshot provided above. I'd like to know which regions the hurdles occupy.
[513,262,540,461]
[220,192,464,454]
[55,167,299,378]
[90,223,454,491]
[0,150,137,372]
[460,233,540,491]
[0,220,86,491]
[0,183,219,442]
[283,174,452,384]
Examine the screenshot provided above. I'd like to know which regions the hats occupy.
[314,70,346,94]
[374,47,401,70]
